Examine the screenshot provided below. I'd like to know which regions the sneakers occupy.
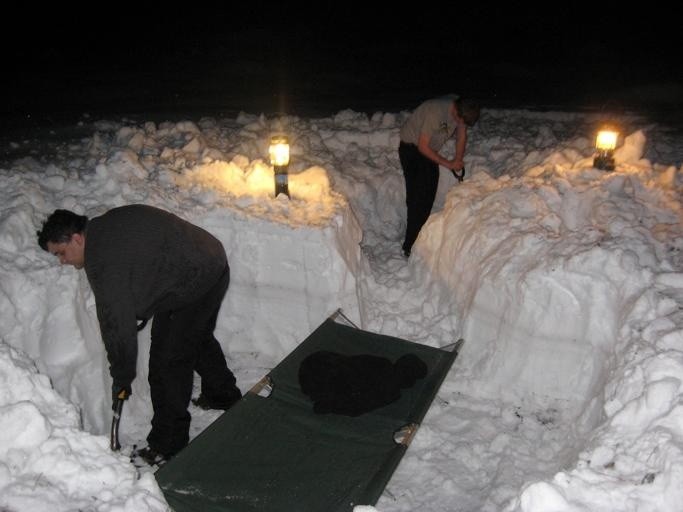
[130,445,170,467]
[192,392,243,410]
[401,242,412,256]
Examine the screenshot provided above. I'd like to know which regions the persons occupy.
[397,91,482,259]
[37,204,243,469]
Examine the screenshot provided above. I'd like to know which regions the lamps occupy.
[593,130,617,171]
[269,134,290,200]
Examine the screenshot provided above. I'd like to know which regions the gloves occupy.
[111,379,132,400]
